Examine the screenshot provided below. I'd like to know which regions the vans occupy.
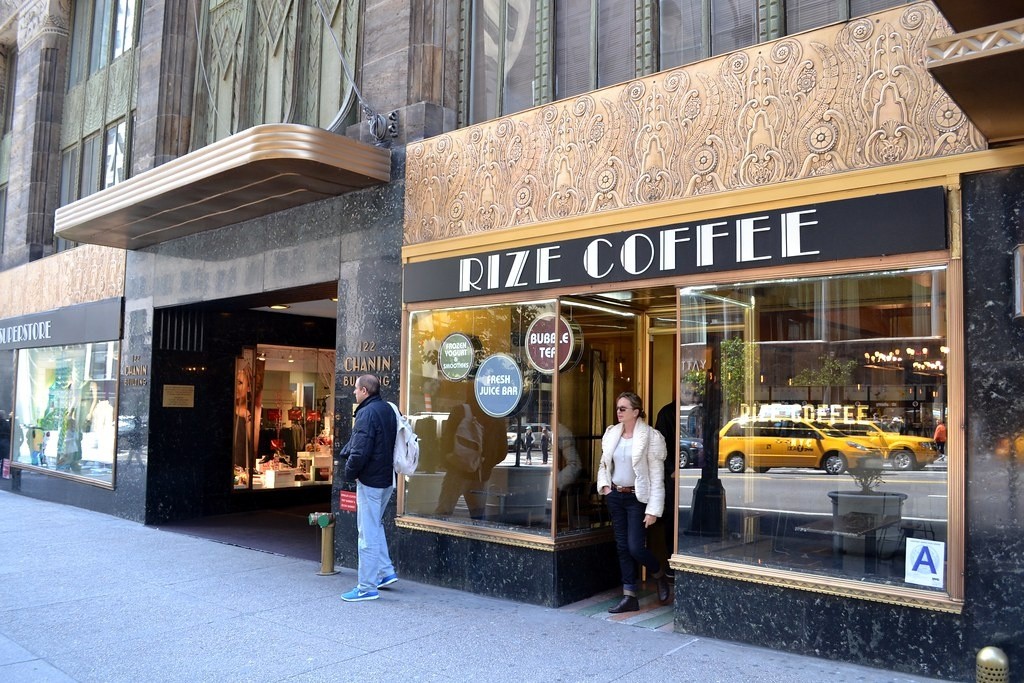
[506,423,552,452]
[719,415,885,478]
[818,418,942,471]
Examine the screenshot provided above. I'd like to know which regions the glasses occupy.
[616,406,632,411]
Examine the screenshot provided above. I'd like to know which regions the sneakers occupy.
[341,586,379,601]
[377,572,399,589]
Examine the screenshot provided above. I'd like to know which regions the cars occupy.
[679,438,705,469]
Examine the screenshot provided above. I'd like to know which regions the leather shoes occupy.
[608,595,639,613]
[654,575,670,602]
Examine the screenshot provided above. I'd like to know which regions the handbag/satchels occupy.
[62,429,78,454]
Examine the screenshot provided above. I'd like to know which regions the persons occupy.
[557,423,582,492]
[934,420,947,455]
[656,401,676,577]
[125,415,145,473]
[71,377,99,432]
[887,417,901,432]
[0,410,11,458]
[338,374,402,601]
[597,391,669,613]
[434,382,508,520]
[234,364,264,471]
[540,427,551,464]
[524,426,535,465]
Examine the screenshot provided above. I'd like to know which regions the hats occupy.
[526,426,532,429]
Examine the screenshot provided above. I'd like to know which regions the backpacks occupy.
[387,401,420,476]
[446,404,485,482]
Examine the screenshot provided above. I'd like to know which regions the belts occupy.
[611,482,635,494]
